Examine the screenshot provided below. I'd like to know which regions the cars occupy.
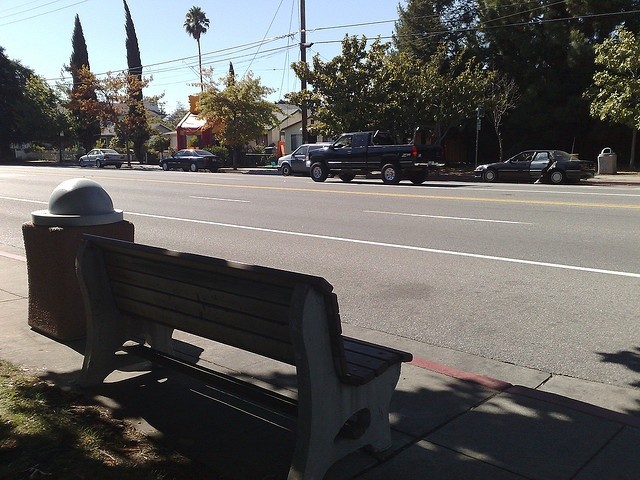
[158,149,217,171]
[474,149,595,184]
[79,149,125,168]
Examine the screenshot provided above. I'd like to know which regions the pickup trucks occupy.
[306,131,445,185]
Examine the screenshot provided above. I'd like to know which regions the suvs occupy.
[278,144,334,176]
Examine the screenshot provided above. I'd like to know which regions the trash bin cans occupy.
[597,147,617,175]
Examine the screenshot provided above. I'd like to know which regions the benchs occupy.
[72,231,413,479]
